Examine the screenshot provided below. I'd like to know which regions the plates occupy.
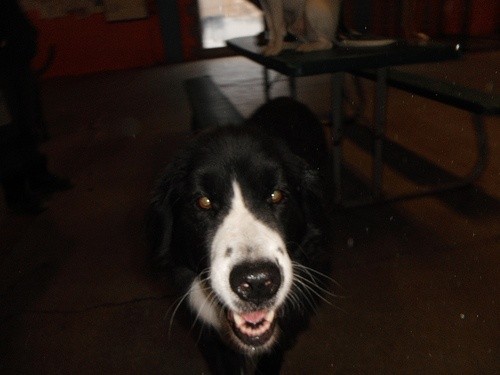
[340,39,396,47]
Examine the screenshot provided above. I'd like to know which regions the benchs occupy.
[183,75,247,129]
[345,67,500,182]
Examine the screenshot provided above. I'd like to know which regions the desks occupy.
[227,34,459,207]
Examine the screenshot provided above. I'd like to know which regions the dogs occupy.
[143,95,349,375]
[244,0,396,57]
